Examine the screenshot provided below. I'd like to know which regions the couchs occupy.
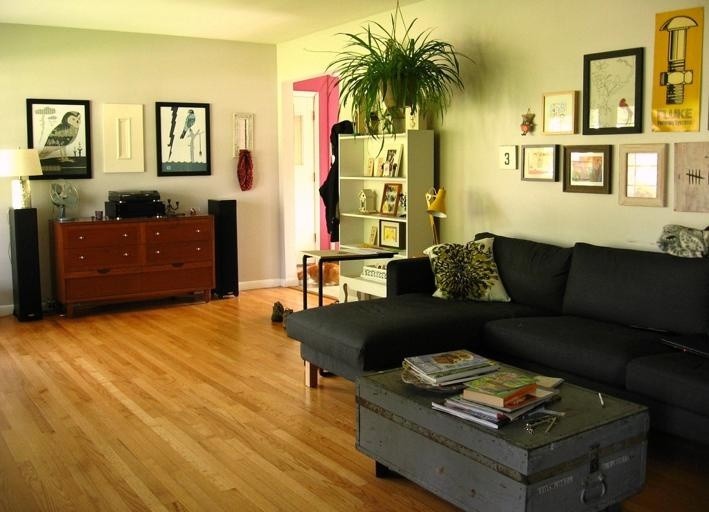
[484,314,709,445]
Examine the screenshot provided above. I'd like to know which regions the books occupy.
[404,349,564,431]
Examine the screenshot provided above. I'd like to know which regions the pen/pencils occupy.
[598,392,605,406]
[522,404,557,433]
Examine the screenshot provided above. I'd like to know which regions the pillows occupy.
[423,237,512,303]
[562,241,709,337]
[475,232,571,314]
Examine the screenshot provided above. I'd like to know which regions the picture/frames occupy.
[26,99,91,179]
[563,145,611,194]
[385,145,403,177]
[101,104,145,174]
[233,112,253,160]
[381,183,401,216]
[618,144,666,207]
[379,219,406,249]
[541,91,578,135]
[583,47,642,134]
[520,144,560,182]
[156,103,211,176]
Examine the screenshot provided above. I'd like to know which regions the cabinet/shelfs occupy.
[338,130,435,305]
[49,215,215,318]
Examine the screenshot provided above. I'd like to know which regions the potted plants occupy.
[307,12,476,157]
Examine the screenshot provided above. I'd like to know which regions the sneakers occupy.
[272,302,293,328]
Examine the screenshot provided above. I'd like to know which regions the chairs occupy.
[287,255,557,388]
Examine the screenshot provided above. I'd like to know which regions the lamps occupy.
[425,186,448,244]
[0,149,43,209]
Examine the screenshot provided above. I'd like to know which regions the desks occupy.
[303,251,394,312]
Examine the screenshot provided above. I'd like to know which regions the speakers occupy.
[209,199,239,298]
[9,208,42,321]
